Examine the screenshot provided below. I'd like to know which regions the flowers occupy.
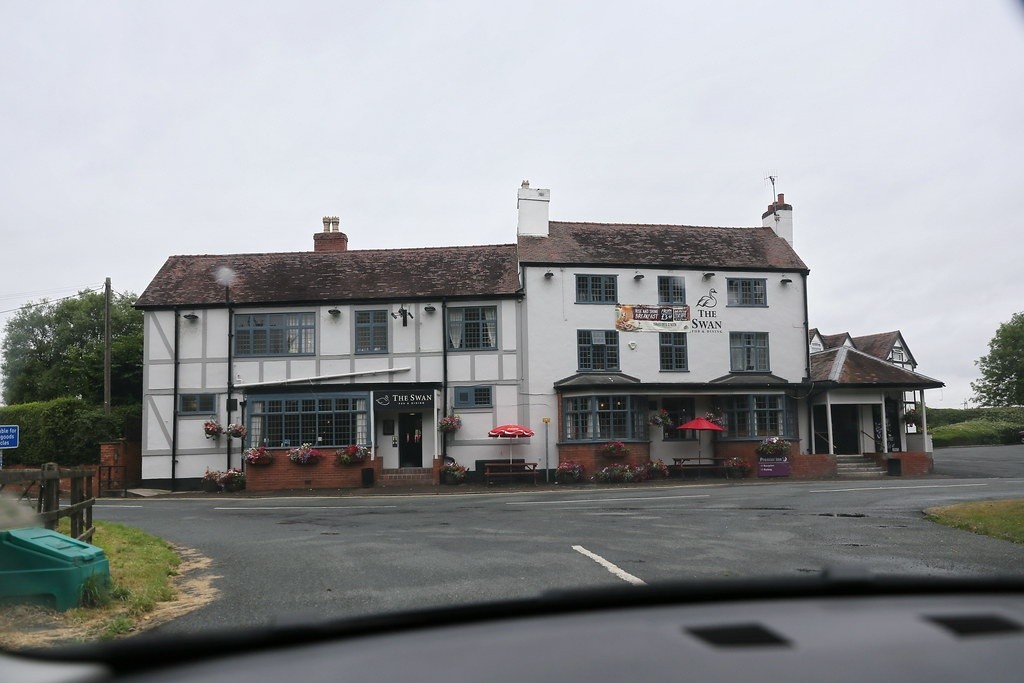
[603,408,727,454]
[905,407,919,425]
[204,406,371,487]
[437,412,466,484]
[555,461,584,482]
[726,431,791,469]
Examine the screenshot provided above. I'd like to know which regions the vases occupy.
[202,430,364,494]
[445,475,459,484]
[604,450,630,457]
[730,467,747,478]
[558,473,574,485]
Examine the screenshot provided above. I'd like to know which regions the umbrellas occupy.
[676,417,725,464]
[488,424,535,472]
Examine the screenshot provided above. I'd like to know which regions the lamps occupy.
[328,306,341,317]
[779,278,792,285]
[183,314,198,321]
[633,271,645,281]
[424,304,435,313]
[544,272,554,280]
[703,272,715,279]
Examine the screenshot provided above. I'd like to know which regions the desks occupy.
[484,463,538,486]
[672,457,731,478]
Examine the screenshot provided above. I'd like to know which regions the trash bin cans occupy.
[0,527,108,611]
[888,459,900,476]
[362,468,373,488]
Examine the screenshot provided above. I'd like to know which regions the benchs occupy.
[669,464,734,470]
[484,471,539,475]
